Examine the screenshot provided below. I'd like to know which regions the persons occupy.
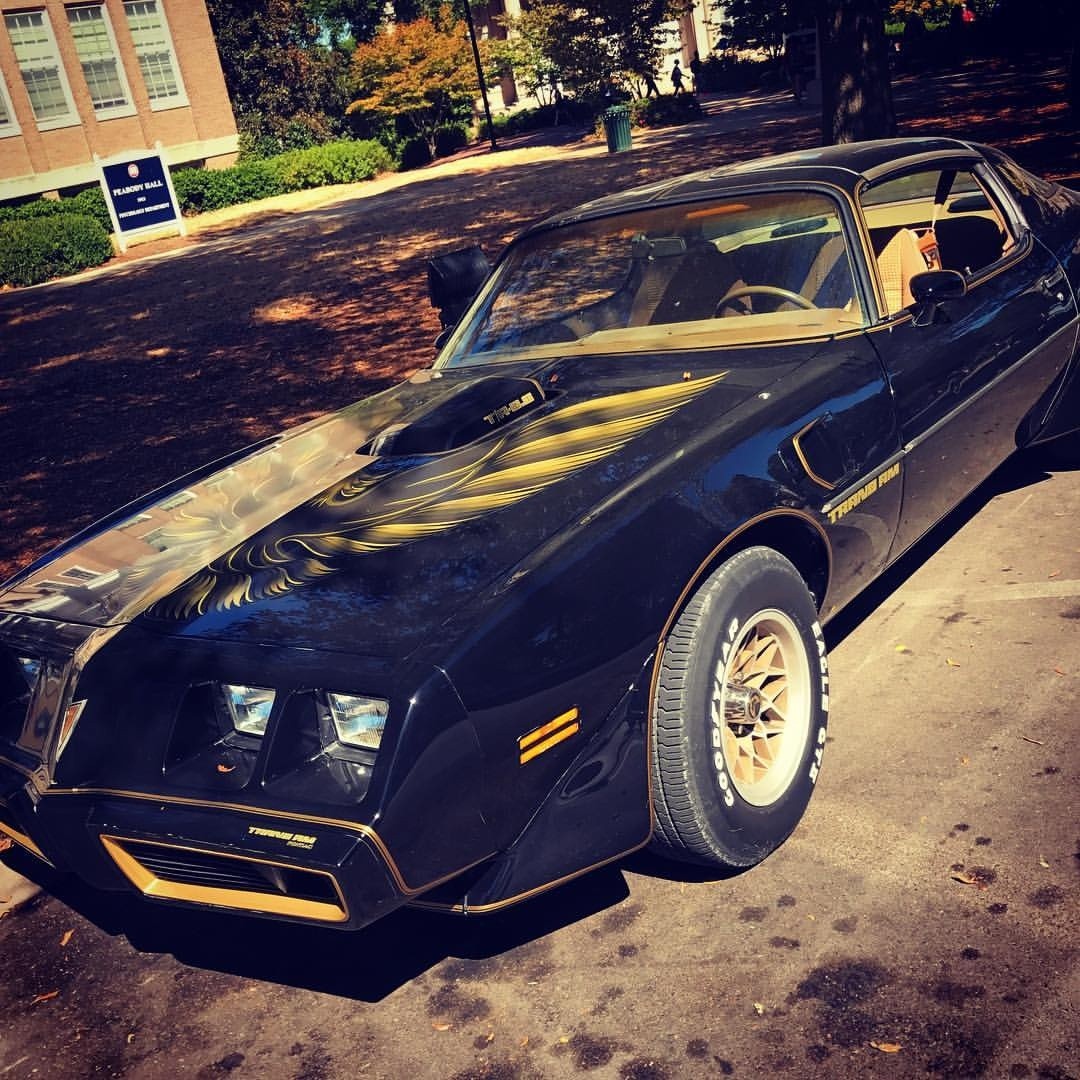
[642,51,701,98]
[897,1,977,80]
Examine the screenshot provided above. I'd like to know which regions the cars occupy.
[1,134,1079,929]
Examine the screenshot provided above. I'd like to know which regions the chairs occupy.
[626,252,752,327]
[797,228,929,317]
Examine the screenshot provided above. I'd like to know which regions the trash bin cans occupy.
[602,105,633,153]
